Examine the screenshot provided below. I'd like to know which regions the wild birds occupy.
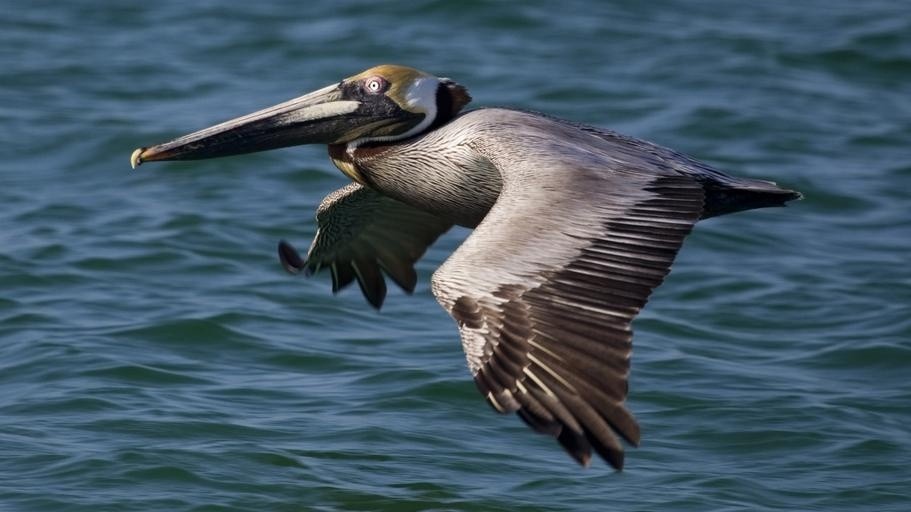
[128,63,804,472]
